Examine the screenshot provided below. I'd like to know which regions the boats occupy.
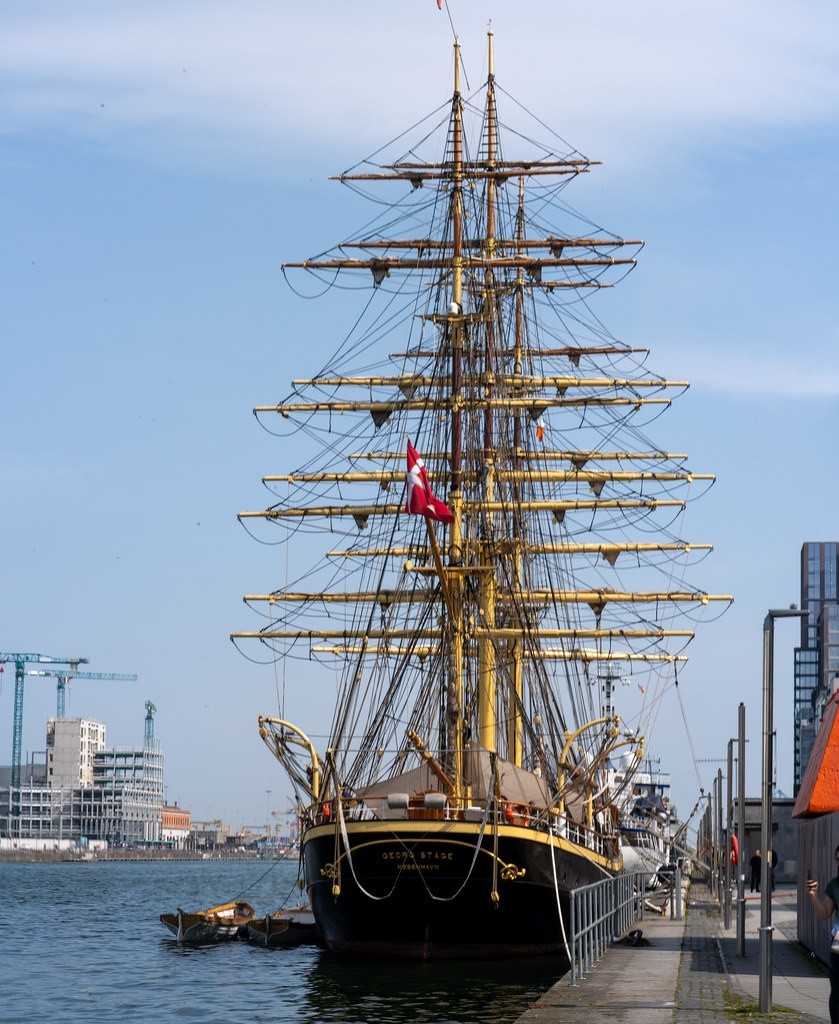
[245,899,316,953]
[158,898,253,949]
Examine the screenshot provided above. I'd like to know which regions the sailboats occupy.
[229,0,734,959]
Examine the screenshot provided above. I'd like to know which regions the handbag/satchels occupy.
[830,931,839,953]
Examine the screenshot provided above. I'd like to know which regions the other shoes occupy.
[756,890,760,892]
[751,890,753,892]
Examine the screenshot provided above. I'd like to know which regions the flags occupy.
[406,440,456,525]
[535,416,546,441]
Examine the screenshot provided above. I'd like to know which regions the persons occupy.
[749,849,761,893]
[805,847,839,1024]
[768,847,778,892]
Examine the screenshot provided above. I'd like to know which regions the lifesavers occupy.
[725,834,739,865]
[505,803,529,826]
[315,803,330,824]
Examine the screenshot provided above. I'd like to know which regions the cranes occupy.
[20,669,137,718]
[144,699,157,749]
[1,651,89,791]
